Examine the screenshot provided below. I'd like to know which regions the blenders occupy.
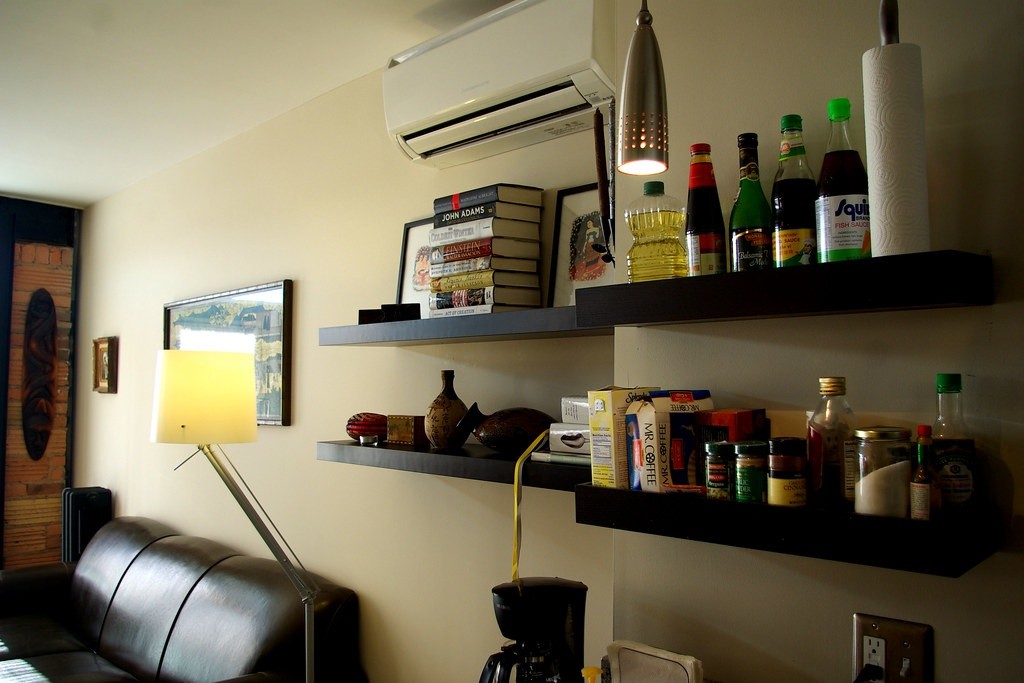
[478,577,588,683]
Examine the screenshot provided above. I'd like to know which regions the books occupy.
[428,183,544,319]
[531,452,592,466]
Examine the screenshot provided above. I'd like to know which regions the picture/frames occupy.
[162,278,293,428]
[92,335,118,393]
[548,179,616,308]
[396,216,444,317]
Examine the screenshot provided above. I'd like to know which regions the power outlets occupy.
[852,610,934,683]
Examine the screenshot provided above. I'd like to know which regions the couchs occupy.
[0,515,354,683]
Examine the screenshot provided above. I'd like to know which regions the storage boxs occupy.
[587,383,664,487]
[627,385,705,496]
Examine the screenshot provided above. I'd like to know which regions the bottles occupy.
[729,133,772,271]
[704,441,733,500]
[910,425,936,524]
[771,115,817,268]
[808,377,855,500]
[932,374,976,509]
[768,437,807,506]
[624,182,690,284]
[856,426,911,515]
[817,98,871,264]
[684,143,726,277]
[735,442,767,501]
[424,370,469,448]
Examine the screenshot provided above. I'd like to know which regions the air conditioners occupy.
[382,0,614,173]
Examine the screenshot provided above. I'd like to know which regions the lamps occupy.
[617,0,669,174]
[152,350,315,683]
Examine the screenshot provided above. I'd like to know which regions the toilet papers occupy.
[860,40,929,257]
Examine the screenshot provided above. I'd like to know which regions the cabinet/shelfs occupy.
[316,306,611,491]
[573,248,1023,577]
